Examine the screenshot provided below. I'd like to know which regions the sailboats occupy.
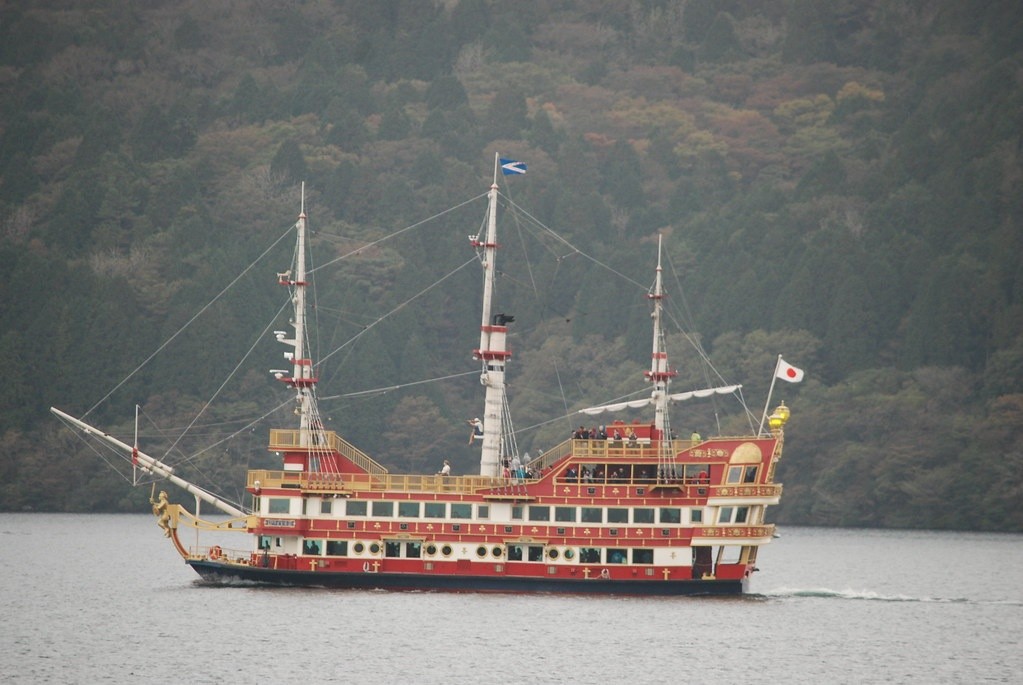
[49,150,791,593]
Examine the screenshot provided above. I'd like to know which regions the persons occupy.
[566,466,578,482]
[501,448,552,479]
[634,468,709,484]
[468,418,484,445]
[438,460,451,490]
[572,425,638,447]
[691,432,701,446]
[581,467,605,483]
[610,468,628,483]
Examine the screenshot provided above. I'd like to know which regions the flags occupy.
[776,360,804,383]
[501,159,527,175]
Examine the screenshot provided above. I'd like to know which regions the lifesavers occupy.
[209,545,222,561]
